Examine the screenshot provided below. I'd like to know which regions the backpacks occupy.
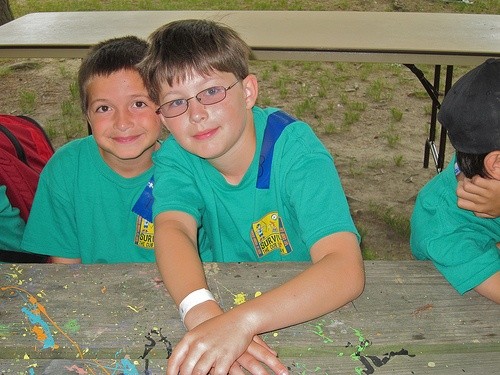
[0,114,55,224]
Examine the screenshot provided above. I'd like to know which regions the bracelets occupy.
[179,288,219,330]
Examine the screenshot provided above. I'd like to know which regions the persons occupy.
[135,18,366,375]
[410,57,500,306]
[21,36,218,264]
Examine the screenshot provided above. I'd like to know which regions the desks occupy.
[0,10,500,175]
[0,260,500,375]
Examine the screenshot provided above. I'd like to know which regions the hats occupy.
[437,58,500,153]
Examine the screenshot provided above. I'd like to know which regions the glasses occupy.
[155,77,244,118]
